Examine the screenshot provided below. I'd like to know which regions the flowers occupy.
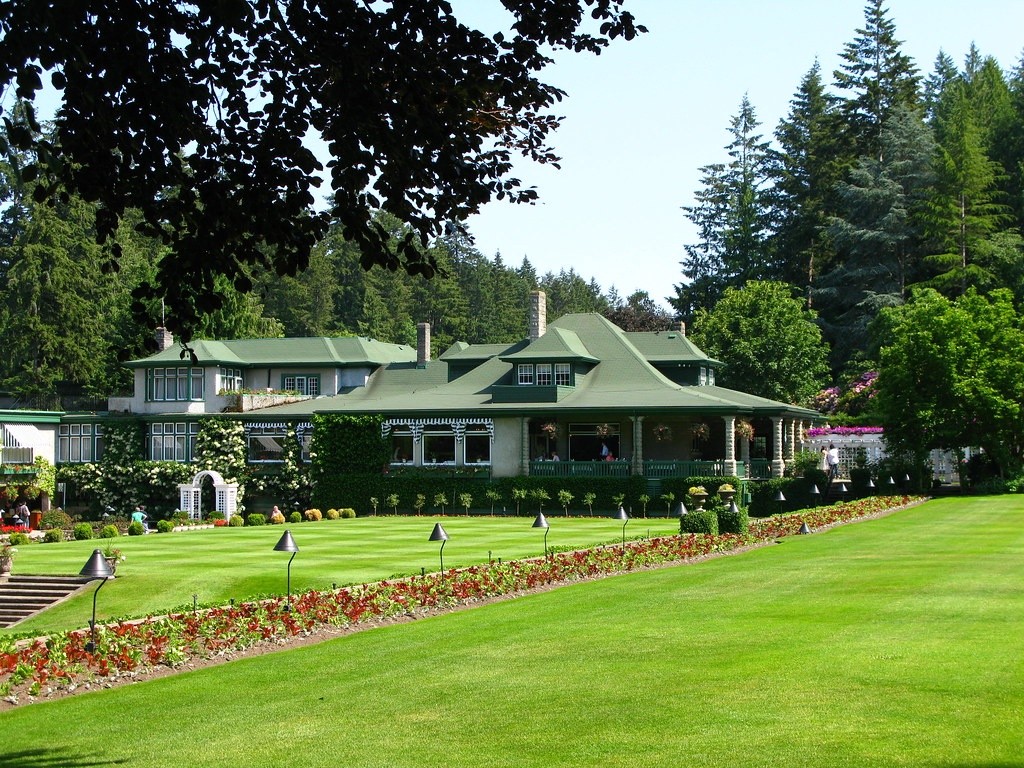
[0,542,18,566]
[1,463,36,473]
[595,421,616,439]
[687,422,710,441]
[735,418,755,442]
[24,481,41,500]
[1,481,19,503]
[170,518,214,527]
[653,423,674,442]
[689,484,708,495]
[718,483,735,491]
[541,418,561,440]
[104,547,125,564]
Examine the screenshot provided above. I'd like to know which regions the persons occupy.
[606,452,614,462]
[16,501,30,528]
[600,442,608,461]
[821,443,838,478]
[551,451,560,461]
[131,506,149,534]
[535,455,543,461]
[271,506,281,518]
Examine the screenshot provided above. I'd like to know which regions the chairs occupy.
[534,457,723,475]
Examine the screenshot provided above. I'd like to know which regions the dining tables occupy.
[695,464,719,475]
[650,464,676,469]
[611,464,629,470]
[534,464,554,470]
[576,464,592,471]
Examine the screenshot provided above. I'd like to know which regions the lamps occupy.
[1,509,24,526]
[887,476,895,498]
[427,523,451,585]
[839,483,848,506]
[866,478,876,500]
[727,501,739,514]
[674,501,689,538]
[532,511,551,564]
[102,505,115,520]
[273,530,300,605]
[57,506,62,511]
[79,549,115,657]
[613,505,629,551]
[809,484,820,513]
[903,474,911,481]
[775,491,787,525]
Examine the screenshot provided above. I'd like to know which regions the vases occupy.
[689,494,709,513]
[0,555,12,576]
[0,467,37,475]
[717,491,737,508]
[171,524,216,532]
[104,557,117,580]
